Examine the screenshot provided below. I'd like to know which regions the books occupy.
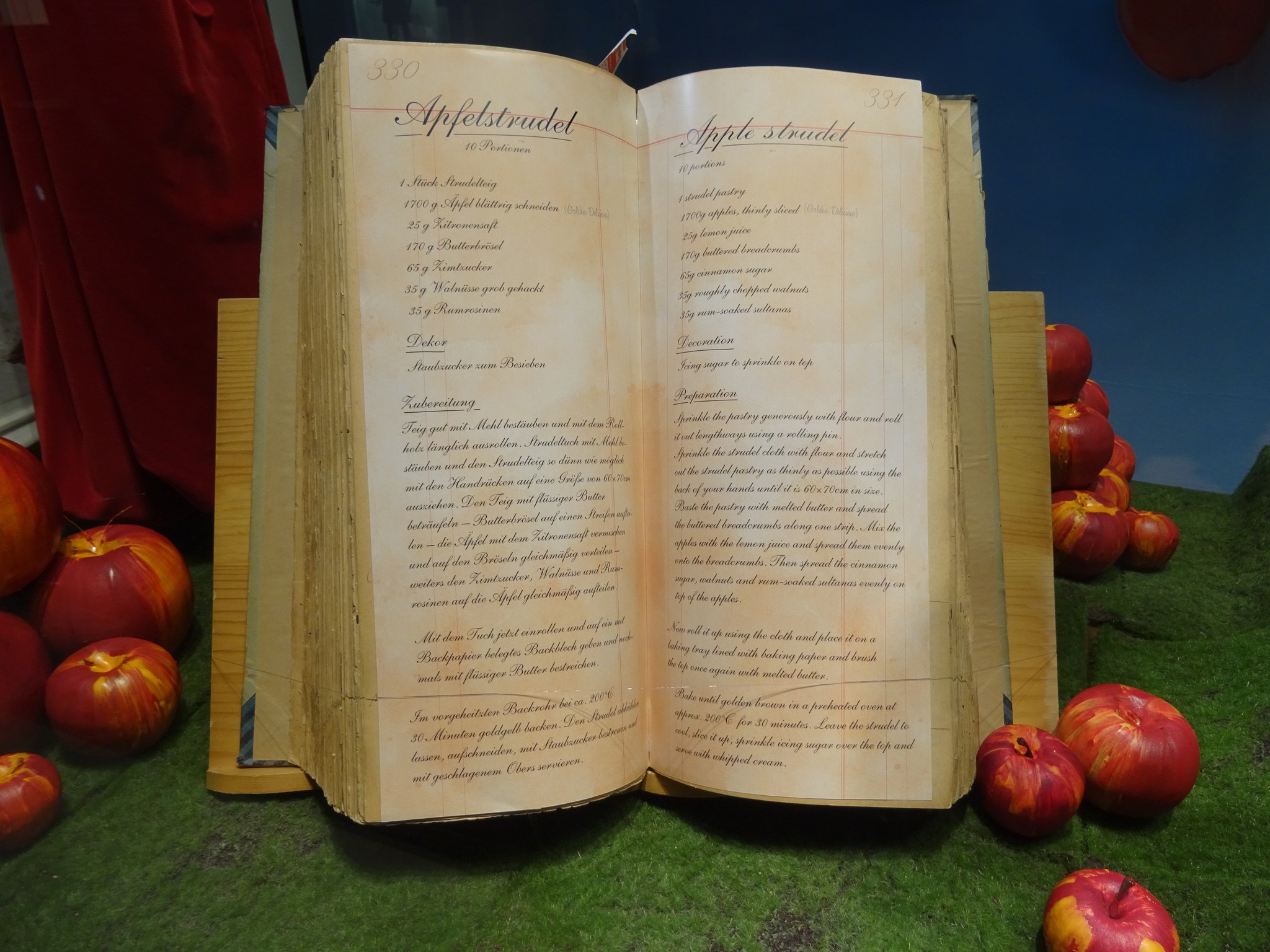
[233,29,1015,837]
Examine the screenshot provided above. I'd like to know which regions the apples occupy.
[1039,865,1183,952]
[1042,312,1187,566]
[1,429,64,599]
[974,716,1084,842]
[23,519,196,651]
[45,631,180,745]
[0,748,59,846]
[1057,683,1201,815]
[0,613,52,732]
